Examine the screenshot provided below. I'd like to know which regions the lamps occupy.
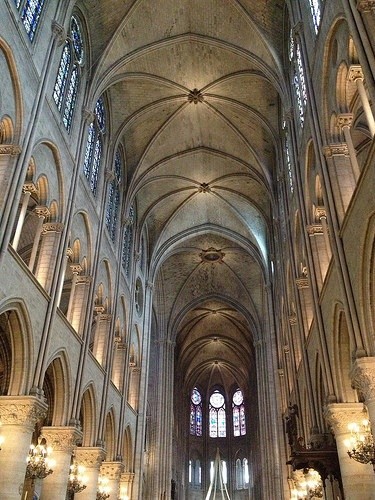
[25,444,54,489]
[96,477,111,500]
[117,487,129,500]
[342,420,375,472]
[68,465,88,499]
[291,470,323,500]
